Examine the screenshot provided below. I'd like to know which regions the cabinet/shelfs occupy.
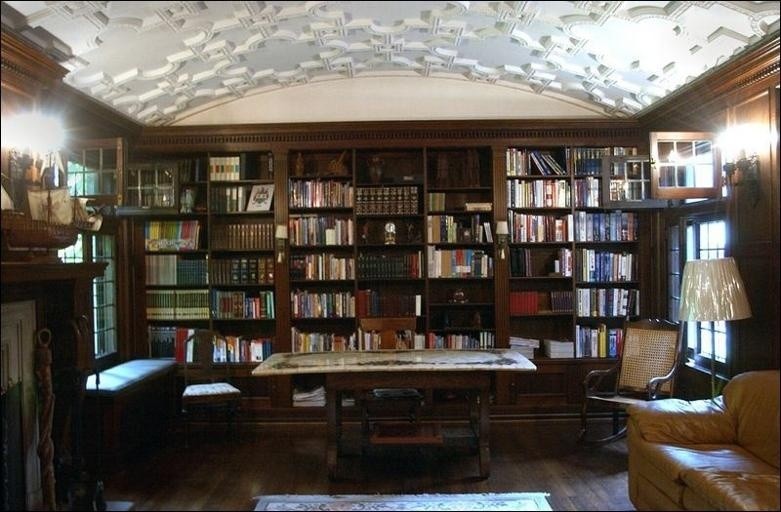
[118,120,680,419]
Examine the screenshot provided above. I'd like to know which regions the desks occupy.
[251,349,538,483]
[86,357,175,473]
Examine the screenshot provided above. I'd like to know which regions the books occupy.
[506,147,574,358]
[574,148,641,358]
[135,157,210,362]
[427,151,495,350]
[293,383,495,407]
[355,156,426,350]
[209,153,275,363]
[288,180,356,353]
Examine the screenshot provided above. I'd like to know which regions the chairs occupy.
[579,317,680,447]
[181,328,243,450]
[360,317,423,434]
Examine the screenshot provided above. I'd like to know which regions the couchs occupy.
[628,369,780,511]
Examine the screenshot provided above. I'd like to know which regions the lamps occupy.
[680,257,752,399]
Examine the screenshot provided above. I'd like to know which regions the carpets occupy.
[255,492,551,511]
